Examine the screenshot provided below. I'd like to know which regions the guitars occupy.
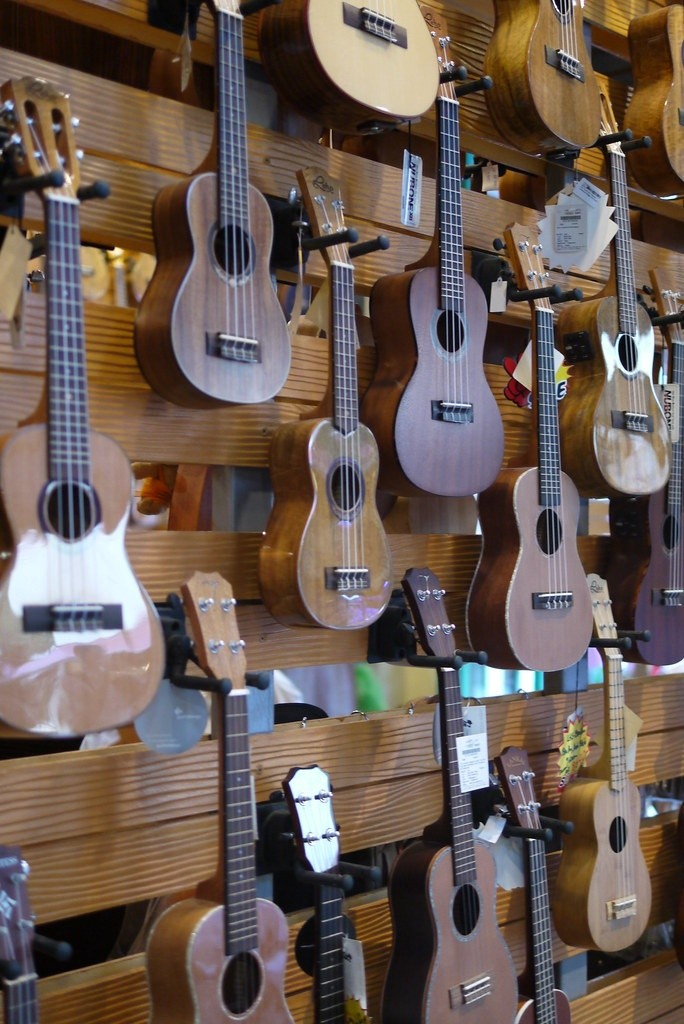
[146,567,297,1024]
[0,74,169,735]
[494,744,571,1024]
[623,1,684,203]
[378,566,518,1023]
[132,0,290,405]
[258,0,441,138]
[280,762,349,1023]
[559,81,672,496]
[554,573,653,953]
[602,266,684,669]
[465,222,595,674]
[360,37,503,499]
[260,163,394,630]
[484,0,602,155]
[0,845,42,1023]
